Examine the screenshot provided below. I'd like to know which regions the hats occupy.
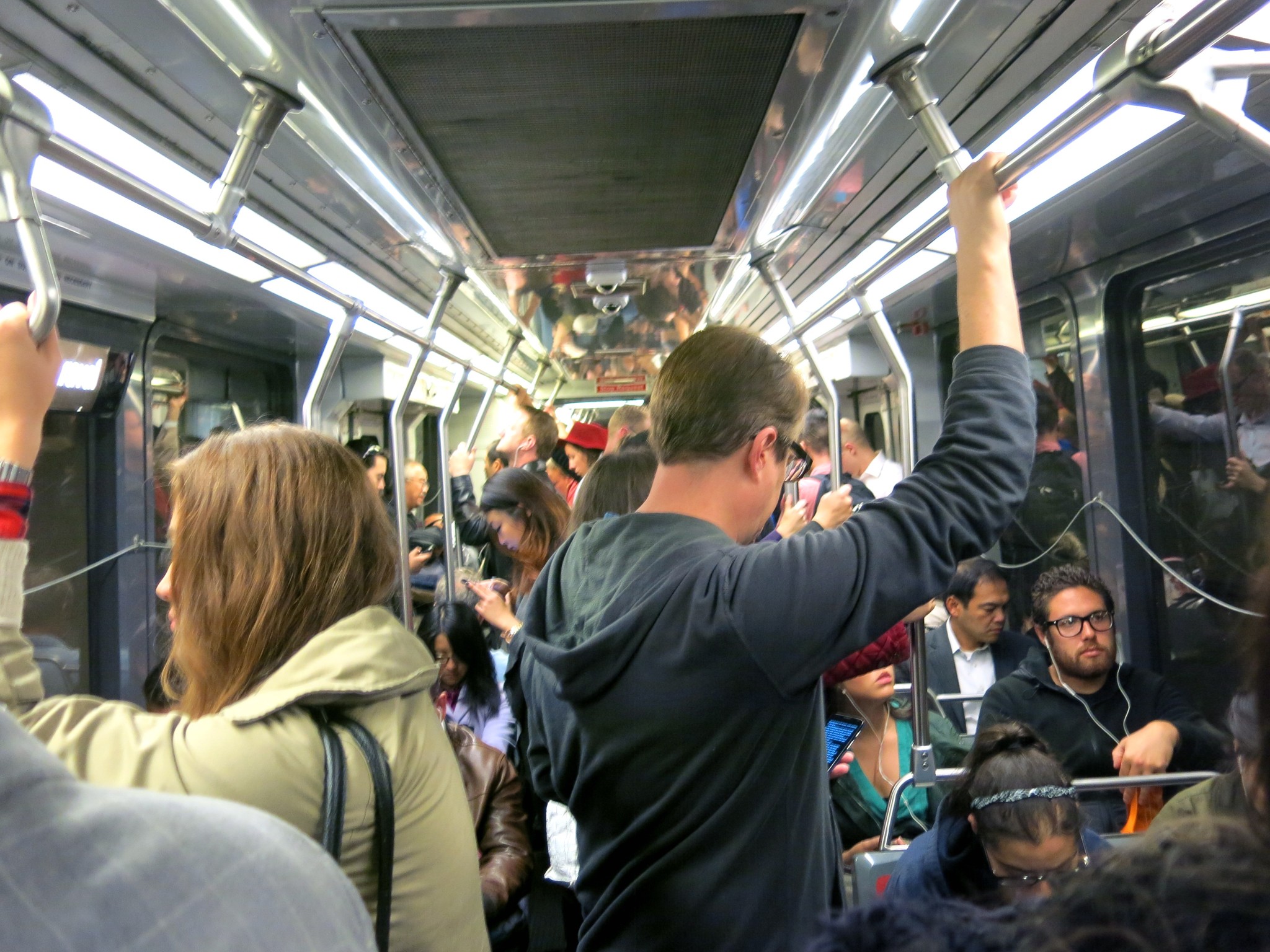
[556,421,608,451]
[1181,365,1222,402]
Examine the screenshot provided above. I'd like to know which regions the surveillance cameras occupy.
[584,270,627,295]
[592,295,629,314]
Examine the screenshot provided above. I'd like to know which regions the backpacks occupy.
[812,474,875,525]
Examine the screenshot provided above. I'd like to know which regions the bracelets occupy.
[0,459,34,488]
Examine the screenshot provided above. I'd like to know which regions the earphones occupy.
[1044,635,1049,648]
[517,442,528,450]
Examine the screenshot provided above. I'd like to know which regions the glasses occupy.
[752,433,814,482]
[981,831,1093,888]
[436,653,460,665]
[1045,609,1115,637]
[360,445,382,462]
[1217,371,1251,401]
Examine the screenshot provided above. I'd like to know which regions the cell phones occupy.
[460,579,468,585]
[823,712,864,774]
[420,544,435,553]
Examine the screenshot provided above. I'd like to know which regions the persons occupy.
[971,563,1233,835]
[1144,688,1270,840]
[884,718,1116,905]
[502,147,1037,952]
[144,386,949,952]
[19,565,87,695]
[0,703,376,952]
[929,557,1033,736]
[1003,344,1270,709]
[0,291,496,952]
